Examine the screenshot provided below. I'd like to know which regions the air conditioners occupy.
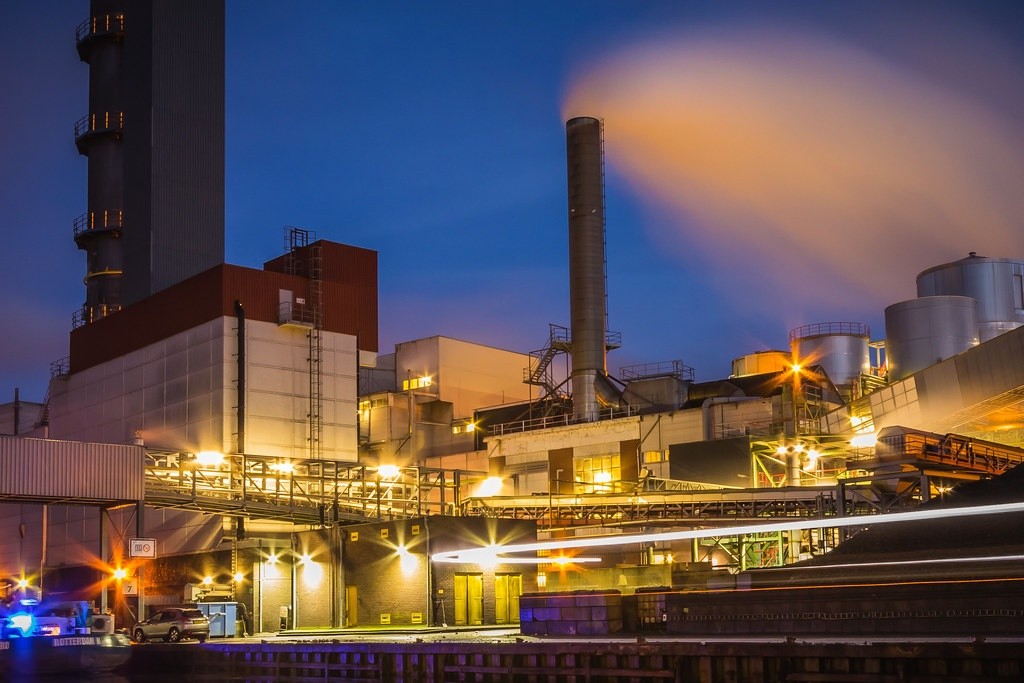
[91,615,111,632]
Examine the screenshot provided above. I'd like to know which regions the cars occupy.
[132,608,210,642]
